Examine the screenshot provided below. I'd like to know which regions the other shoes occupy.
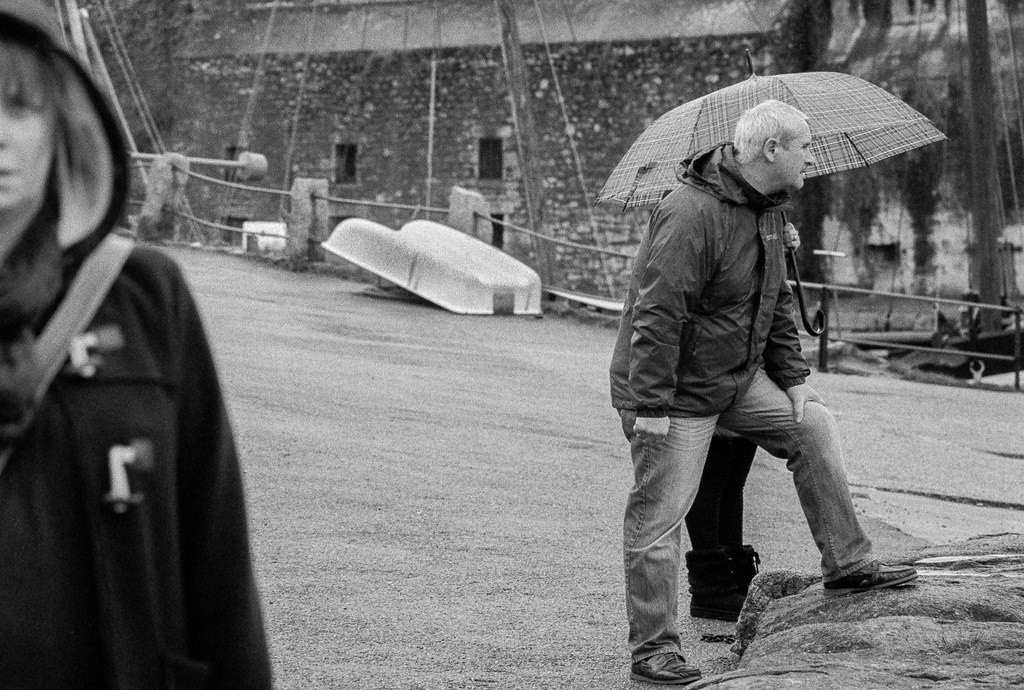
[822,559,919,596]
[630,651,702,684]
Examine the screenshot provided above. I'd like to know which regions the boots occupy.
[684,545,761,622]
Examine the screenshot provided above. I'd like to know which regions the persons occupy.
[609,100,919,683]
[685,222,800,623]
[0,0,274,690]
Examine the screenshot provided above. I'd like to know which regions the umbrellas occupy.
[592,49,953,336]
